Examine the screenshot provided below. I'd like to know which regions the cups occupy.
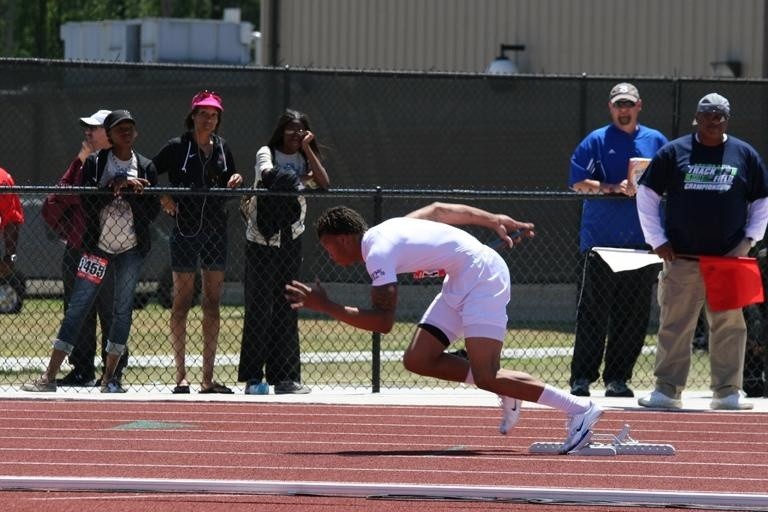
[249,383,269,394]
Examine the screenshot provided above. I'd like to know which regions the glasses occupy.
[614,100,635,108]
[284,129,306,137]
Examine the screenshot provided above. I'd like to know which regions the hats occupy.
[609,82,640,104]
[192,94,224,112]
[79,110,113,129]
[103,110,136,128]
[697,93,730,113]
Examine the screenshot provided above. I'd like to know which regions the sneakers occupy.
[639,391,683,409]
[19,367,127,393]
[499,395,521,435]
[571,382,590,396]
[709,390,753,409]
[558,400,603,455]
[173,381,310,394]
[606,382,633,397]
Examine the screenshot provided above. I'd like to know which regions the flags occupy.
[700,254,765,312]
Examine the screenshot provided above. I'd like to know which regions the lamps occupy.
[488,41,525,76]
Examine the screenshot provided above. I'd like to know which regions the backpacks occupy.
[238,146,309,228]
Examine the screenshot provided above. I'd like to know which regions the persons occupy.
[638,91,766,413]
[149,91,243,393]
[284,199,603,456]
[236,107,329,396]
[18,109,159,392]
[566,81,667,400]
[0,168,24,272]
[41,109,128,386]
